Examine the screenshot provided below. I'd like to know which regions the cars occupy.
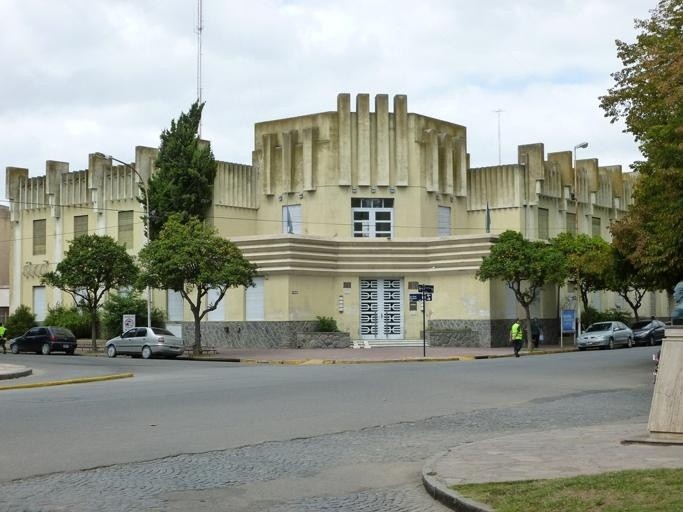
[103,325,186,363]
[630,319,667,346]
[9,325,78,356]
[576,320,636,351]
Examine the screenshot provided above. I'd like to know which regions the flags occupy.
[287,208,294,234]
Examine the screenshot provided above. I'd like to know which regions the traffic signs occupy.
[408,284,434,302]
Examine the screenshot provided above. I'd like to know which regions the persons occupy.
[532,317,543,348]
[0,322,8,354]
[509,318,523,357]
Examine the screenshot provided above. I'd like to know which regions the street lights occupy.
[572,141,591,341]
[94,150,153,329]
